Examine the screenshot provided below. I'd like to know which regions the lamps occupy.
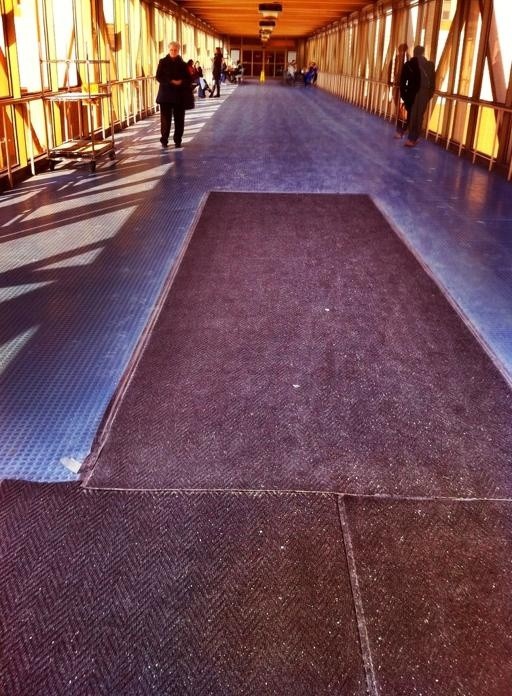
[258,2,282,41]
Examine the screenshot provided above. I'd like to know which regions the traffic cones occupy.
[260,70,265,80]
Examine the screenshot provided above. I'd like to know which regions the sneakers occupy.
[209,94,220,98]
[404,139,421,147]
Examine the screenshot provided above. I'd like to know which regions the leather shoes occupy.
[161,142,182,148]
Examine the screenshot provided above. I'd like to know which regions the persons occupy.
[153,39,187,150]
[188,58,212,98]
[399,45,436,146]
[194,60,202,77]
[208,46,223,97]
[285,59,318,88]
[220,59,243,84]
